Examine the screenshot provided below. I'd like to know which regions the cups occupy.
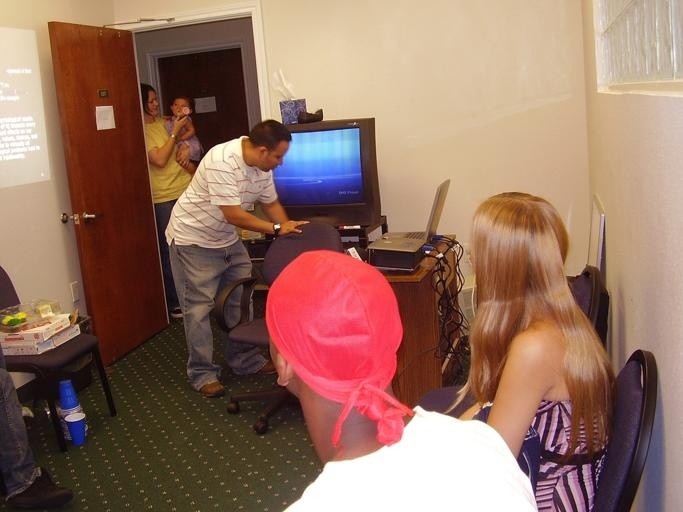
[59,380,88,444]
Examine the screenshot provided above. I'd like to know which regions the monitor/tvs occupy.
[267,116,384,224]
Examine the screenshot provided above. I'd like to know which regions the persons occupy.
[266,249,537,511]
[442,192,617,511]
[140,83,193,319]
[1,367,73,512]
[162,96,204,181]
[164,119,310,398]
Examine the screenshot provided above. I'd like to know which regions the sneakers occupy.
[257,362,278,375]
[171,307,184,319]
[5,475,74,511]
[199,380,225,398]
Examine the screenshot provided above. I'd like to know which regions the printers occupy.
[366,177,454,270]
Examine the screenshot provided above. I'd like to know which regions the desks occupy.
[380,235,464,411]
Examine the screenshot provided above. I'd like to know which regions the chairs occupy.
[591,348,659,511]
[0,264,117,452]
[212,221,345,434]
[421,264,602,418]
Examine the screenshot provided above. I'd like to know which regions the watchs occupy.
[273,223,281,240]
[169,133,178,140]
